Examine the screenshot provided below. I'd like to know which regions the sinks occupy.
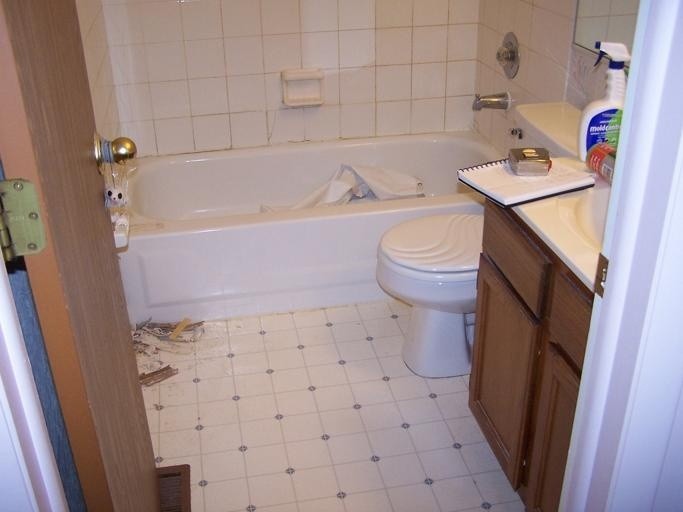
[556,173,612,253]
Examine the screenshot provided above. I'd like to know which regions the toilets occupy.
[375,100,583,380]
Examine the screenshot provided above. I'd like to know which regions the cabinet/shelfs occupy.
[465,201,595,511]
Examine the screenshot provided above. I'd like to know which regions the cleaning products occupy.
[580,39,631,182]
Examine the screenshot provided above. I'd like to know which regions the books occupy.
[457,158,595,209]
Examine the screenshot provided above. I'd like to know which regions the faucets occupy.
[471,92,510,111]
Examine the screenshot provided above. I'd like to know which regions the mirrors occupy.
[572,2,642,69]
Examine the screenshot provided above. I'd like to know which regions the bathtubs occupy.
[116,129,512,327]
